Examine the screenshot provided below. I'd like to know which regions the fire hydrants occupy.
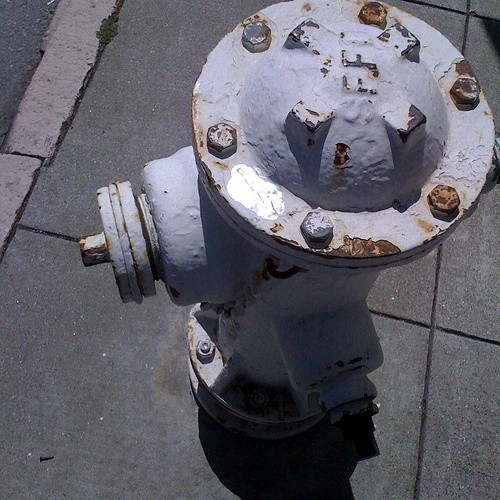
[79,0,500,440]
